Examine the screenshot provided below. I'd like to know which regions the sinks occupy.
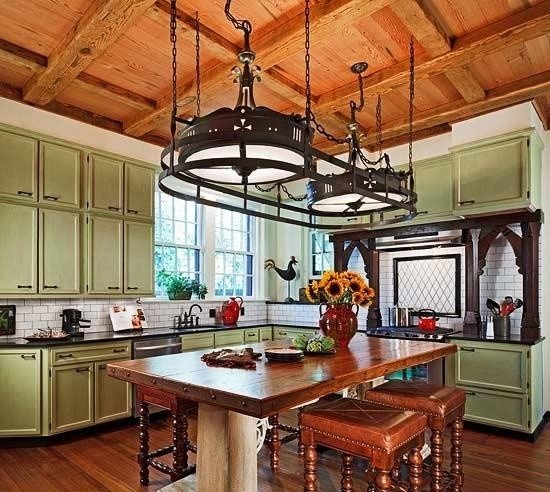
[169,325,223,330]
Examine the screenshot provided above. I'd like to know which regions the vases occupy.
[317,307,360,350]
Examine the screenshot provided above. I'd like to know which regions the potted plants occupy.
[156,268,207,300]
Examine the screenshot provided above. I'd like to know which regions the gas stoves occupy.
[366,325,463,341]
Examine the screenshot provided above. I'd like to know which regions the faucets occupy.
[174,304,202,327]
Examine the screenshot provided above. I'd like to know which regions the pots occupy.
[386,301,415,327]
[59,308,92,334]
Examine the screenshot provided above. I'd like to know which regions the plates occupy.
[23,334,70,341]
[288,345,337,356]
[264,348,304,361]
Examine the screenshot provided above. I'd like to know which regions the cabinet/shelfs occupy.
[259,329,272,341]
[124,221,154,295]
[125,163,154,217]
[181,333,214,352]
[87,150,124,214]
[87,216,124,295]
[455,346,531,395]
[38,138,81,207]
[95,358,131,425]
[376,154,455,226]
[52,342,132,361]
[244,329,259,342]
[456,137,529,206]
[214,331,244,346]
[0,347,41,438]
[273,326,319,343]
[0,129,36,198]
[313,214,373,228]
[51,361,94,435]
[456,387,530,434]
[38,208,82,295]
[1,204,36,295]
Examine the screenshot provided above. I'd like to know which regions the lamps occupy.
[174,0,313,185]
[307,62,402,215]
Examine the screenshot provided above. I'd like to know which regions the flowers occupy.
[306,272,375,307]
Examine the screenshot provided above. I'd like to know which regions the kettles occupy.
[419,309,440,331]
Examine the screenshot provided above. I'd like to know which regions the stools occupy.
[363,379,466,492]
[264,412,300,473]
[133,383,196,486]
[298,396,428,492]
